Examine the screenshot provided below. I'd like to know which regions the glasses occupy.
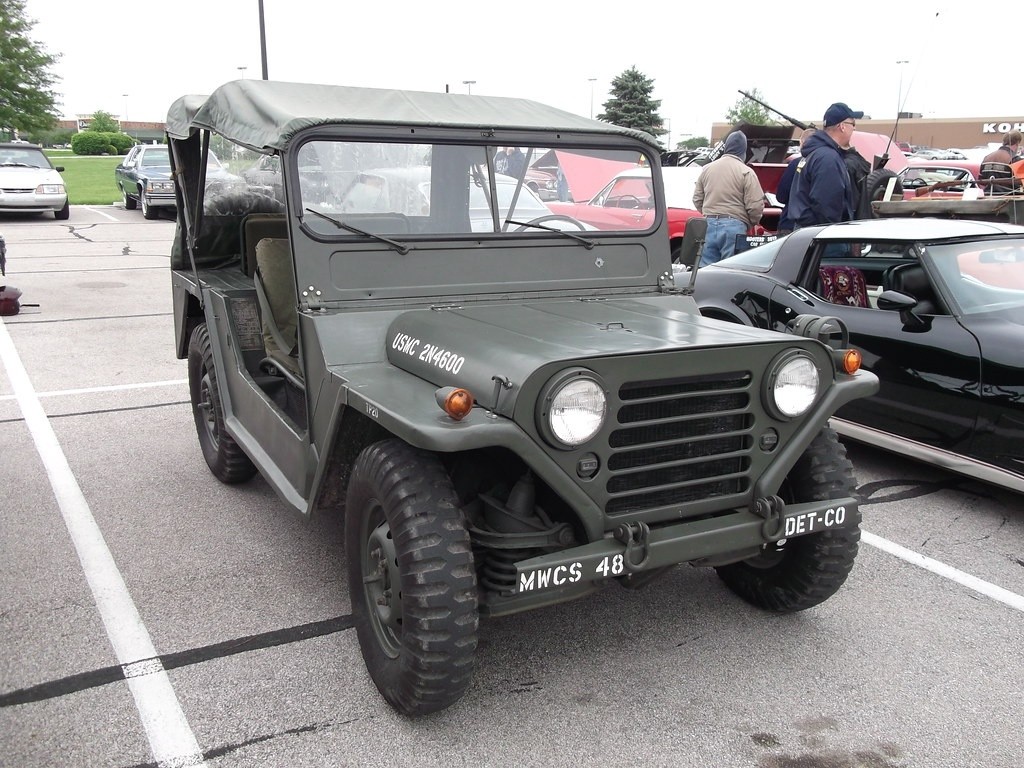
[836,120,855,127]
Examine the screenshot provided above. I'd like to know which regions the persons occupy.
[692,130,766,267]
[980,131,1022,164]
[775,103,873,257]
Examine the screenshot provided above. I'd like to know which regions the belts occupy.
[705,215,732,218]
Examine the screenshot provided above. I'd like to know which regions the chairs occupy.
[815,265,872,309]
[882,260,956,314]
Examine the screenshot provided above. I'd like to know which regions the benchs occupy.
[241,213,410,276]
[252,237,304,385]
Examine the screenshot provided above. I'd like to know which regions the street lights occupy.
[589,78,597,118]
[464,80,477,94]
[123,94,129,122]
[895,59,909,119]
[237,66,248,80]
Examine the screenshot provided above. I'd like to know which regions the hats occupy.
[823,102,864,126]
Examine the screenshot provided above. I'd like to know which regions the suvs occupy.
[165,79,880,717]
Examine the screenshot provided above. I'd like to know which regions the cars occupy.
[0,142,69,220]
[115,145,246,220]
[673,217,1024,493]
[244,119,1024,262]
